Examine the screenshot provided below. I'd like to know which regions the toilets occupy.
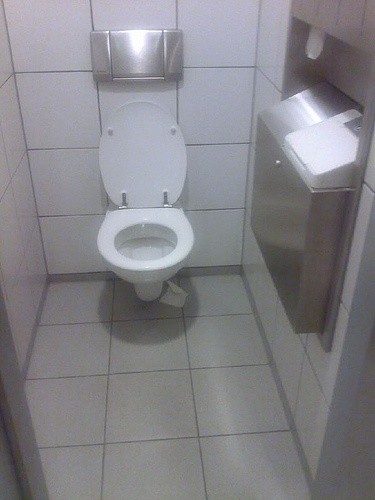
[95,99,196,302]
